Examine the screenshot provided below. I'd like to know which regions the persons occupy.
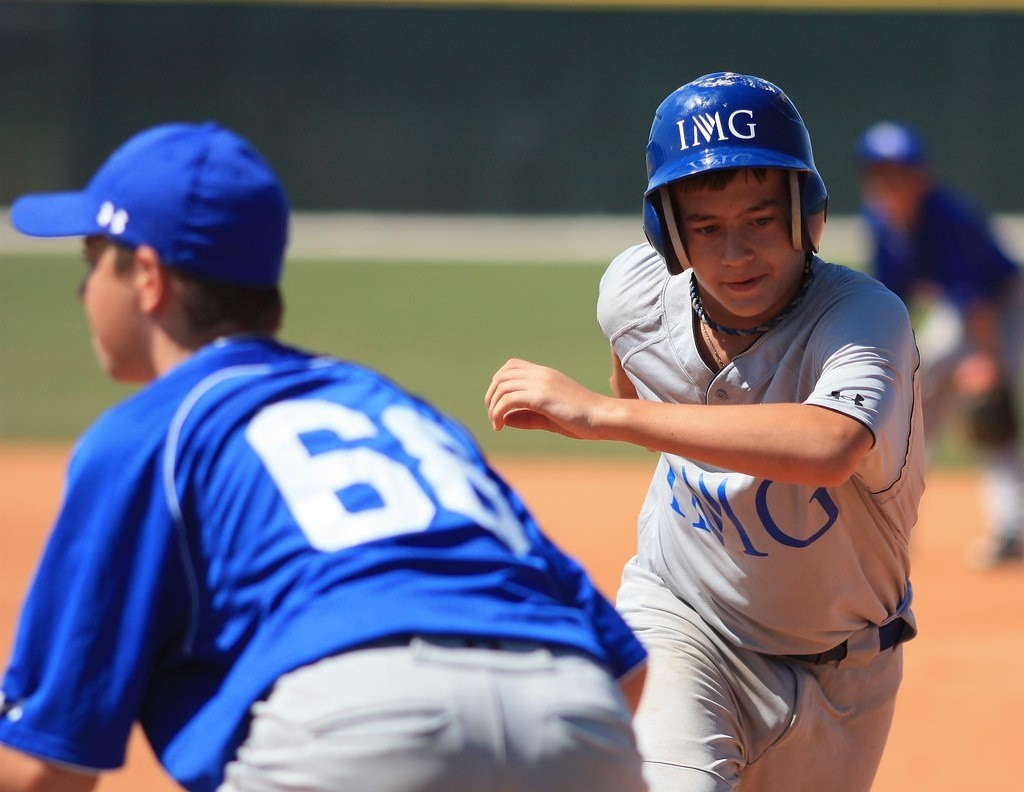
[484,70,926,792]
[849,121,1024,562]
[0,117,648,791]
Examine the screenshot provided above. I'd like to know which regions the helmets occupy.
[642,71,829,275]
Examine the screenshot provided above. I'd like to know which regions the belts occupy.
[758,616,917,665]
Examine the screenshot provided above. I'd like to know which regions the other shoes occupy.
[984,464,1024,558]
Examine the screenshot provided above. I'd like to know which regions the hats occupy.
[858,121,926,165]
[10,121,289,283]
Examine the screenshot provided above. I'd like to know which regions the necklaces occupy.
[689,271,815,335]
[702,316,791,367]
